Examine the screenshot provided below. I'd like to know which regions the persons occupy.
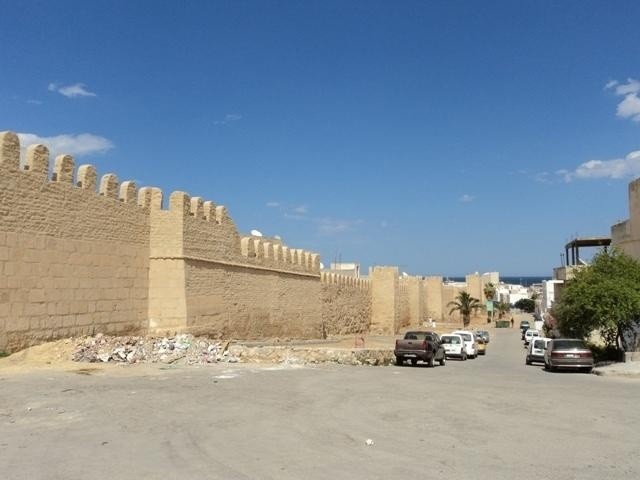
[511,317,515,328]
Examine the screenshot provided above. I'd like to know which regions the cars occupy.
[440,329,490,361]
[521,320,594,372]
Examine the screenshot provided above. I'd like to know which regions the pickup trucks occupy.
[393,330,447,366]
